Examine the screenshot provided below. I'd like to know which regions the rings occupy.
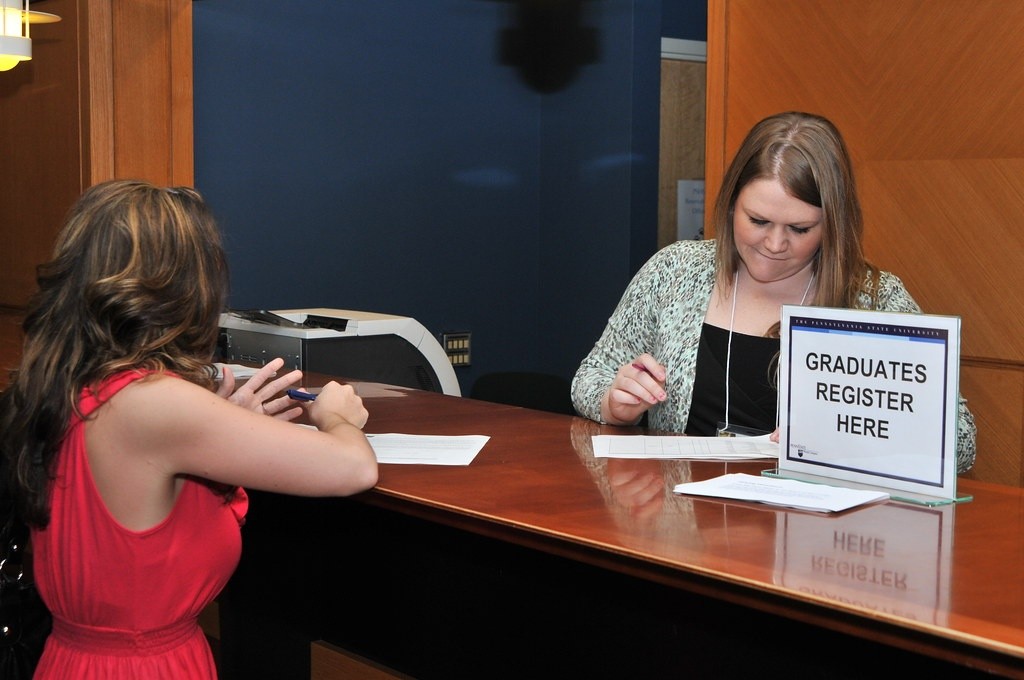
[263,402,271,416]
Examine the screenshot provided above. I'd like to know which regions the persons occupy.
[570,416,774,575]
[569,111,977,477]
[0,177,380,679]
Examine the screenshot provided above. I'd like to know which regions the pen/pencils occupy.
[286,389,319,402]
[632,363,648,372]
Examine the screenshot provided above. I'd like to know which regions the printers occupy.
[217,306,461,400]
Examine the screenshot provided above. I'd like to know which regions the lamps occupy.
[0,0,63,72]
